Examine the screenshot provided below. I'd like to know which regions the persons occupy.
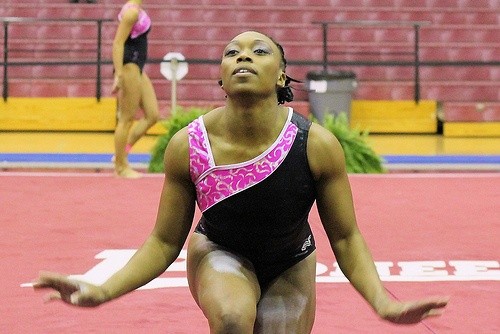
[110,0,162,180]
[29,30,451,334]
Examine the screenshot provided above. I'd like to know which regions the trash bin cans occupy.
[304,70,360,137]
